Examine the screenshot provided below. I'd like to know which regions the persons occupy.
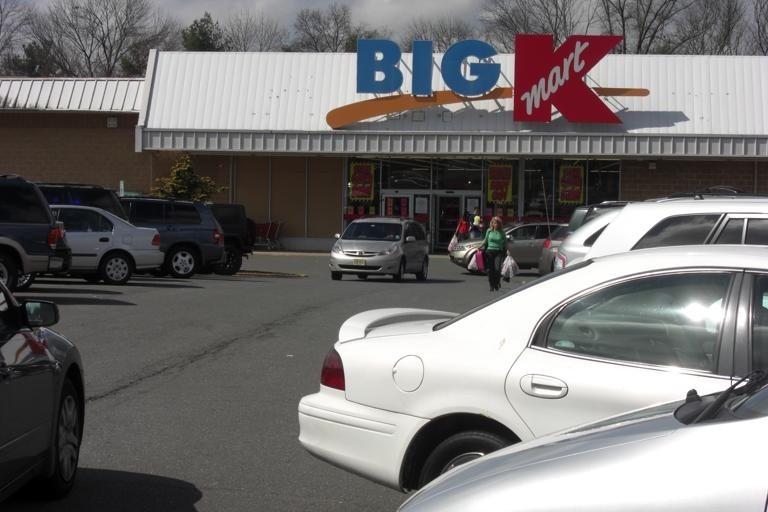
[453,211,469,242]
[477,215,507,293]
[468,207,483,240]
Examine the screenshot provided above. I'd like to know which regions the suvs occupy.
[539,184,768,325]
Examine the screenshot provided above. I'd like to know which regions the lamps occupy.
[648,160,657,171]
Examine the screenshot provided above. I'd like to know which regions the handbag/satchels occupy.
[477,248,488,271]
[503,277,511,283]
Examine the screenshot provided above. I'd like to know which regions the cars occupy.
[1,279,84,511]
[0,176,256,291]
[449,219,560,275]
[297,243,768,495]
[395,369,768,512]
[328,214,430,283]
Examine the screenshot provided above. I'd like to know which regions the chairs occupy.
[252,222,280,253]
